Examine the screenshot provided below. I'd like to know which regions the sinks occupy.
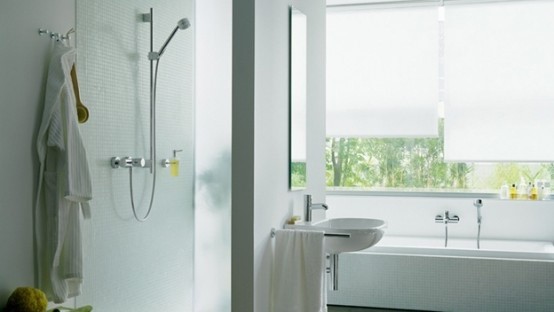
[315,216,387,254]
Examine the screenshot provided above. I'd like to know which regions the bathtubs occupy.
[323,236,550,311]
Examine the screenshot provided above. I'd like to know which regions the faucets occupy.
[302,195,329,221]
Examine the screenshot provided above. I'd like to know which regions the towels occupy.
[268,229,328,312]
[36,36,93,304]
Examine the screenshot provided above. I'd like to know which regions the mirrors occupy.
[290,5,307,192]
